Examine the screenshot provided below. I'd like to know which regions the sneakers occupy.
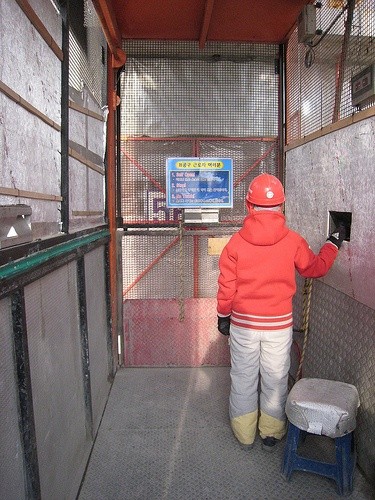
[261,436,279,451]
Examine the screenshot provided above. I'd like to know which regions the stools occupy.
[278,377,364,498]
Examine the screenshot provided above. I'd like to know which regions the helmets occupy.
[246,173,286,207]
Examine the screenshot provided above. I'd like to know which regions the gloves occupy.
[217,315,231,335]
[326,221,346,248]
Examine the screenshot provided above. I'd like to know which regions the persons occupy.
[217,171,346,450]
